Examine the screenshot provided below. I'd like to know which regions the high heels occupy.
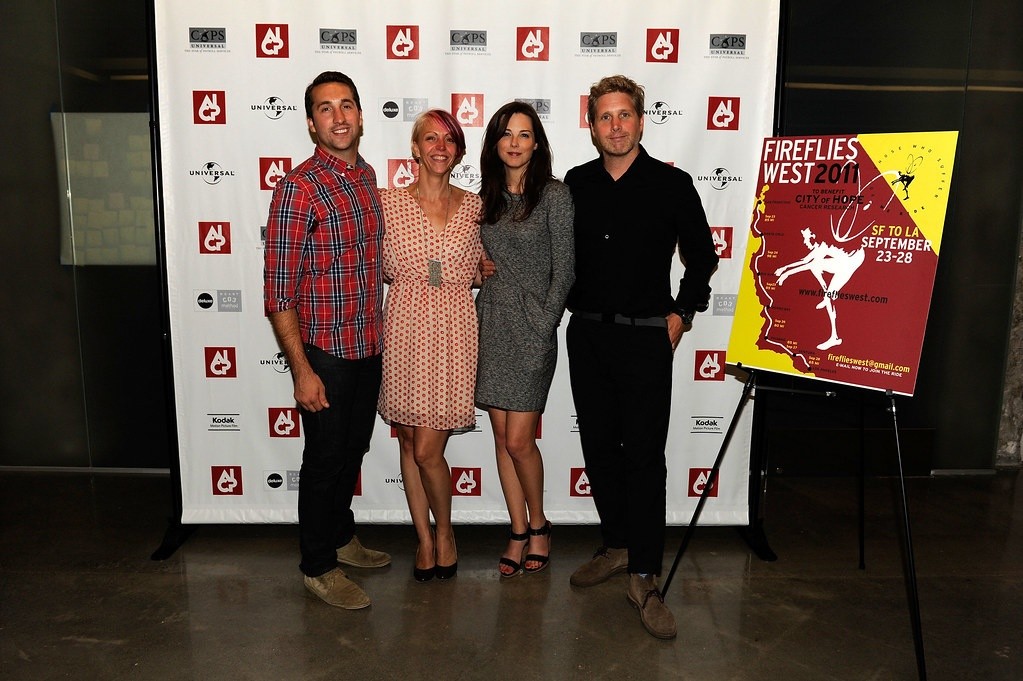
[499,522,530,578]
[523,520,552,574]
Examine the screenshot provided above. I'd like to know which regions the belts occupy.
[575,311,669,329]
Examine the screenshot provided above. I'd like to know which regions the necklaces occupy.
[416,183,451,287]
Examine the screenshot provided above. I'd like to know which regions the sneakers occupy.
[570,546,629,586]
[628,572,678,640]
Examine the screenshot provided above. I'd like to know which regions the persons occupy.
[375,108,482,584]
[263,72,394,609]
[481,75,719,637]
[476,101,577,578]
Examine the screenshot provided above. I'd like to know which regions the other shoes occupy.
[414,528,435,582]
[304,566,371,609]
[336,535,392,568]
[435,529,458,579]
